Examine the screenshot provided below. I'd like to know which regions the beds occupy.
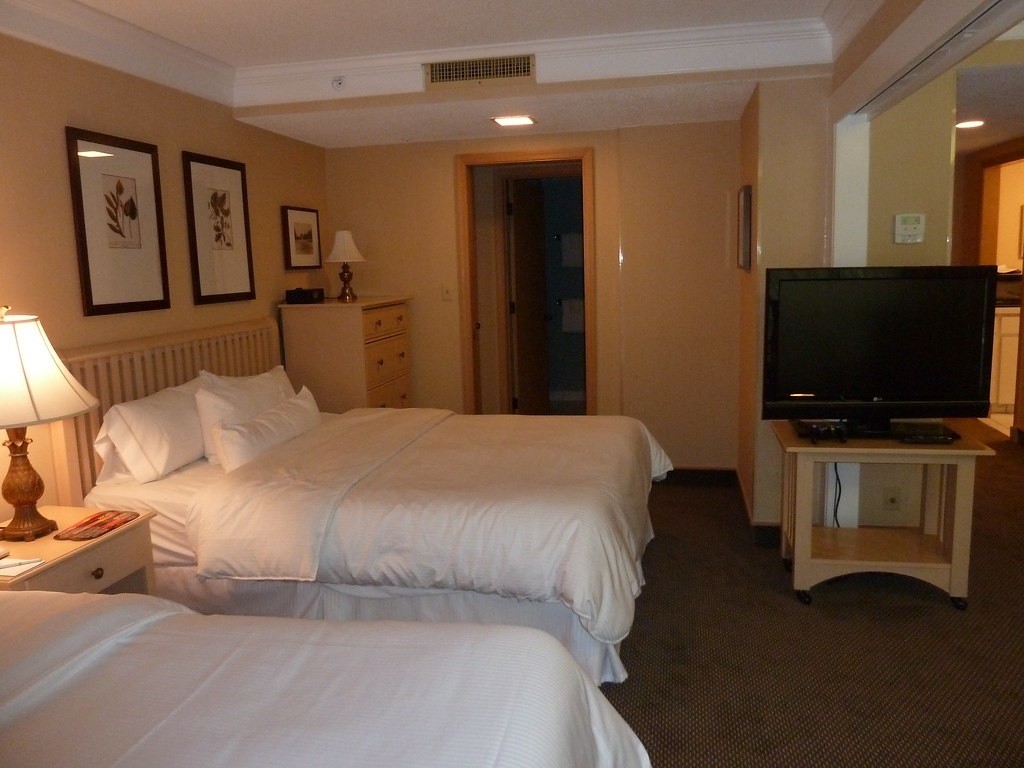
[0,589,653,768]
[49,318,675,687]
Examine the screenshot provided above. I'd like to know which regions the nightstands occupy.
[0,504,158,595]
[279,295,414,416]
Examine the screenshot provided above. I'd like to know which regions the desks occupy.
[771,419,997,608]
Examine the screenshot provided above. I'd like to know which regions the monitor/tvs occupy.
[763,264,998,438]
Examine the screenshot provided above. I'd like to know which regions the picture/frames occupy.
[181,150,256,305]
[281,204,323,270]
[736,184,752,270]
[64,124,172,318]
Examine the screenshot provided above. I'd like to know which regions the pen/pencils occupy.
[0,557,42,569]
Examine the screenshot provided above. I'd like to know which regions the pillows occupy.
[202,370,257,386]
[104,369,216,484]
[212,386,322,474]
[196,365,296,466]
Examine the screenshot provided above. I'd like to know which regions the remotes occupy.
[900,434,953,444]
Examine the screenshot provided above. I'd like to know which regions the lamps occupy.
[0,303,101,542]
[324,231,366,299]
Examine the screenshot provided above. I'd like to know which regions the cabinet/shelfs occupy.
[990,308,1021,413]
